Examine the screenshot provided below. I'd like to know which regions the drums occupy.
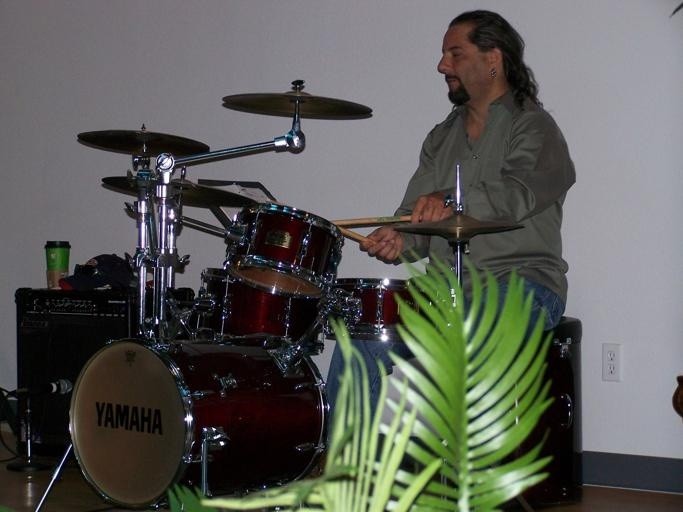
[224,202,345,298]
[69,335,330,507]
[190,269,324,357]
[326,278,456,341]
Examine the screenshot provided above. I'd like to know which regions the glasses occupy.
[74,264,108,282]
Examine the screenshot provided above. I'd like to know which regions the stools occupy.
[409,317,582,506]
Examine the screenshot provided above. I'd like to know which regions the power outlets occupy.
[602,343,621,381]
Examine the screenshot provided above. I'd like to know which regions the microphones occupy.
[8,378,74,399]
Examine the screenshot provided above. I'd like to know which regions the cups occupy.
[45,238,71,290]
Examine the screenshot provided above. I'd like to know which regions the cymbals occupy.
[393,214,526,238]
[100,177,258,208]
[222,92,373,120]
[77,130,210,158]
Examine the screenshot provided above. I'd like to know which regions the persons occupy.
[323,11,578,497]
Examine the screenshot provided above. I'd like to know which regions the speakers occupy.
[14,288,194,457]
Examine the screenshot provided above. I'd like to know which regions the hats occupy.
[58,255,133,291]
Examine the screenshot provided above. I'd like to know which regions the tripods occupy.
[34,152,175,512]
